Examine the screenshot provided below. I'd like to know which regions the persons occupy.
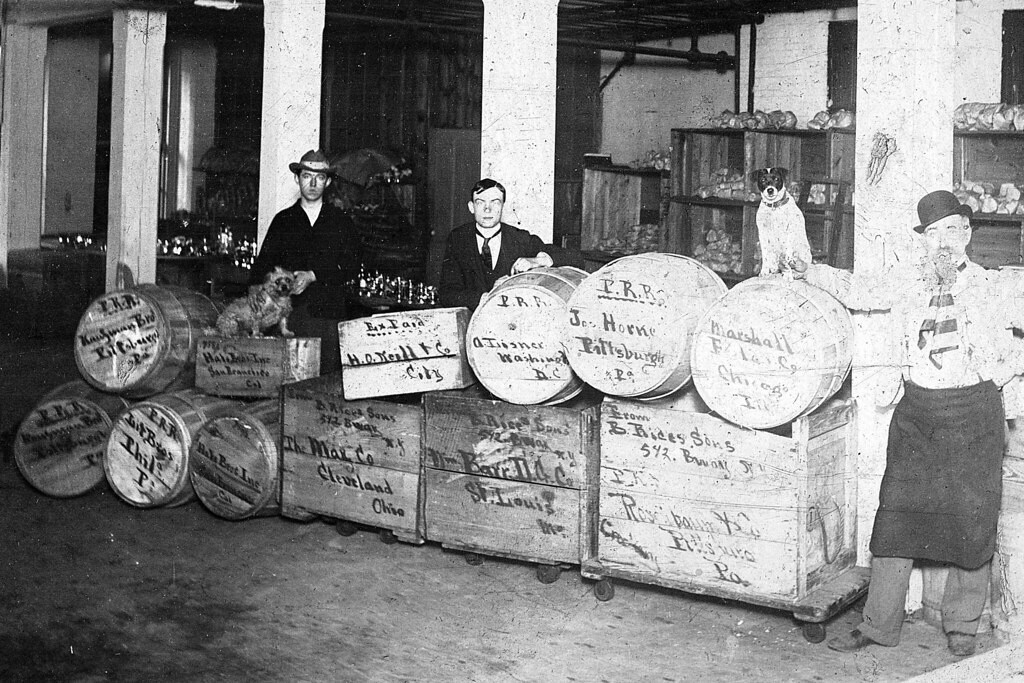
[778,190,1024,656]
[438,179,586,314]
[248,149,365,319]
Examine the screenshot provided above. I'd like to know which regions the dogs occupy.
[746,166,818,287]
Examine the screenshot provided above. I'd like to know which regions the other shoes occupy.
[827,629,877,652]
[948,631,975,655]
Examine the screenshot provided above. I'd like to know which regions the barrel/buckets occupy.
[563,251,728,405]
[102,387,253,510]
[921,558,992,633]
[13,379,141,498]
[188,398,282,521]
[73,285,226,399]
[690,275,853,430]
[465,265,592,409]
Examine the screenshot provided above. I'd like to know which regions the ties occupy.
[475,227,502,270]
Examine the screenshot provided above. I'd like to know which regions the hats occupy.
[289,149,336,178]
[913,190,973,234]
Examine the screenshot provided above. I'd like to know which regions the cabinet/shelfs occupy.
[952,126,1024,271]
[665,123,856,290]
